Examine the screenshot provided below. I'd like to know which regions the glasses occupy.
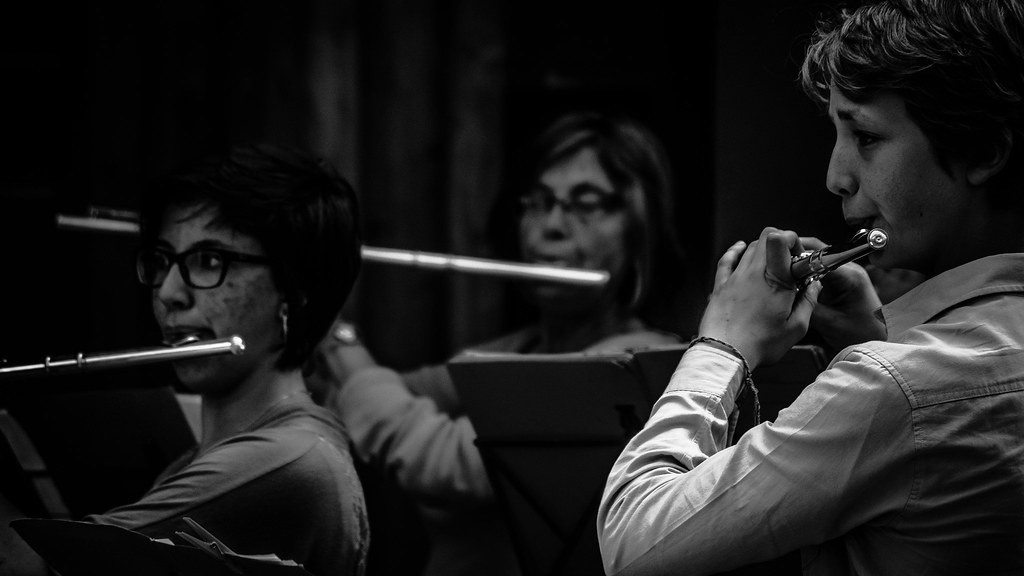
[135,242,280,290]
[519,188,631,225]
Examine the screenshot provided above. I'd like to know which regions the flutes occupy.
[790,227,890,285]
[59,209,611,287]
[0,334,246,384]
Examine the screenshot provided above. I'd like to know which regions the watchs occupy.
[319,322,357,353]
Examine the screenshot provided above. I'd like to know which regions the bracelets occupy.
[689,338,761,426]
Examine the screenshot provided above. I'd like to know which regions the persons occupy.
[0,146,366,576]
[314,113,684,576]
[597,0,1024,576]
